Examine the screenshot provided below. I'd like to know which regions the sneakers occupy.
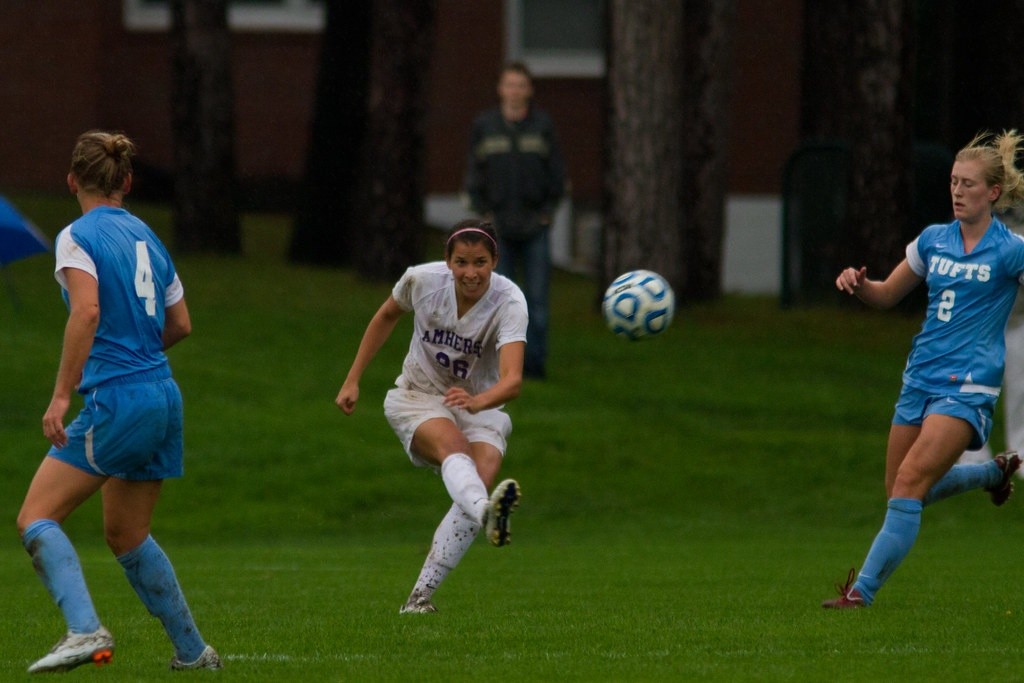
[169,645,222,672]
[485,479,522,547]
[822,567,864,609]
[26,625,114,674]
[400,602,435,613]
[983,452,1023,507]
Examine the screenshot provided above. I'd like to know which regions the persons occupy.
[335,219,528,615]
[821,128,1024,609]
[464,65,564,380]
[16,133,221,673]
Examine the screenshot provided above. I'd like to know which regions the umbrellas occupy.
[0,197,46,262]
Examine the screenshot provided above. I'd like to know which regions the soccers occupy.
[601,268,675,343]
